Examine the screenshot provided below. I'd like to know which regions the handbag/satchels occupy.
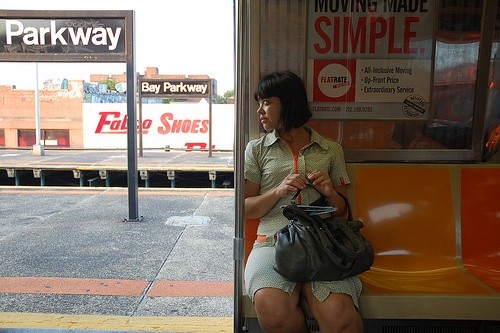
[272,182,375,281]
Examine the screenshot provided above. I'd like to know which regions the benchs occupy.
[240,164,498,320]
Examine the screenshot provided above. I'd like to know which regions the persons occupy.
[243,70,365,333]
